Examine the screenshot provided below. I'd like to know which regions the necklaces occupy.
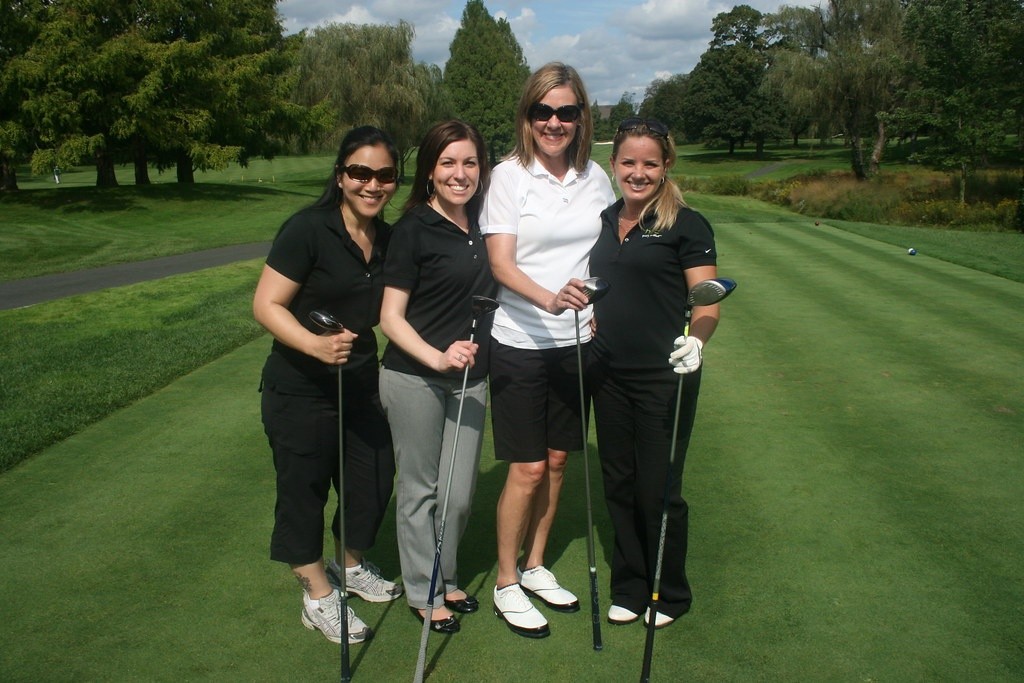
[618,206,638,242]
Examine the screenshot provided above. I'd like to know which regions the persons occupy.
[252,127,403,647]
[478,63,613,638]
[378,120,497,635]
[54,166,61,184]
[587,118,722,625]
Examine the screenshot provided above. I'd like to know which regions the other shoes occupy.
[608,604,637,623]
[644,606,674,628]
[409,601,461,633]
[444,592,479,613]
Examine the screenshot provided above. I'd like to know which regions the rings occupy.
[457,354,463,360]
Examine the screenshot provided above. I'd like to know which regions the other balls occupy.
[909,248,916,255]
[815,221,819,225]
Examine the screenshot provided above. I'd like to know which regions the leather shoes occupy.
[492,585,551,638]
[517,564,581,613]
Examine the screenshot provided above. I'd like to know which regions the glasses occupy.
[527,103,580,123]
[342,164,398,184]
[616,119,668,140]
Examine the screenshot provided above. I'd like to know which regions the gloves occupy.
[668,337,702,374]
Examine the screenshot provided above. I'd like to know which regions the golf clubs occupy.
[574,277,614,650]
[640,278,739,683]
[308,308,351,683]
[413,295,501,683]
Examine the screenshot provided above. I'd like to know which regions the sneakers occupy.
[325,558,403,602]
[301,591,373,644]
